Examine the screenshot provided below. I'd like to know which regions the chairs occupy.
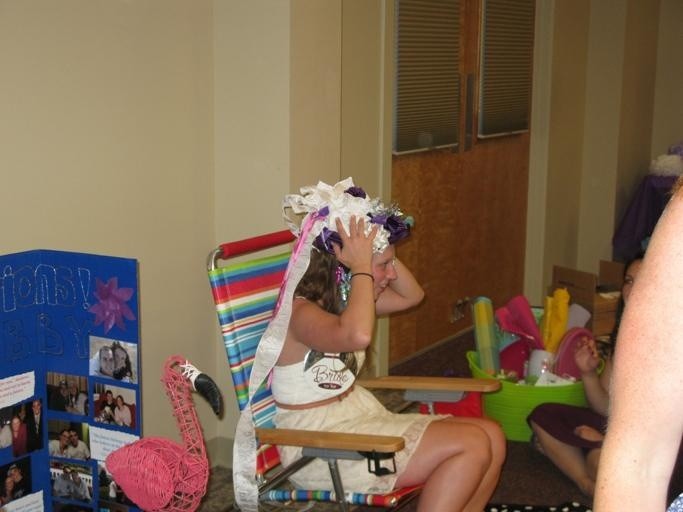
[206,226,503,511]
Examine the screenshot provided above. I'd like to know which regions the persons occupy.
[269,198,508,512]
[592,170,683,512]
[0,340,140,511]
[528,249,646,497]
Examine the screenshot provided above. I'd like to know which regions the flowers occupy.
[281,172,417,258]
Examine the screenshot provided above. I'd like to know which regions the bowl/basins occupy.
[464,352,605,444]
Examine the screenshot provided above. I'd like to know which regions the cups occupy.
[530,349,553,375]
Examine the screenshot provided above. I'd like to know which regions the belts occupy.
[274,382,357,411]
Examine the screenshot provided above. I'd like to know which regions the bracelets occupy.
[351,272,375,281]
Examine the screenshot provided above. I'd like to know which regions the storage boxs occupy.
[551,259,628,339]
